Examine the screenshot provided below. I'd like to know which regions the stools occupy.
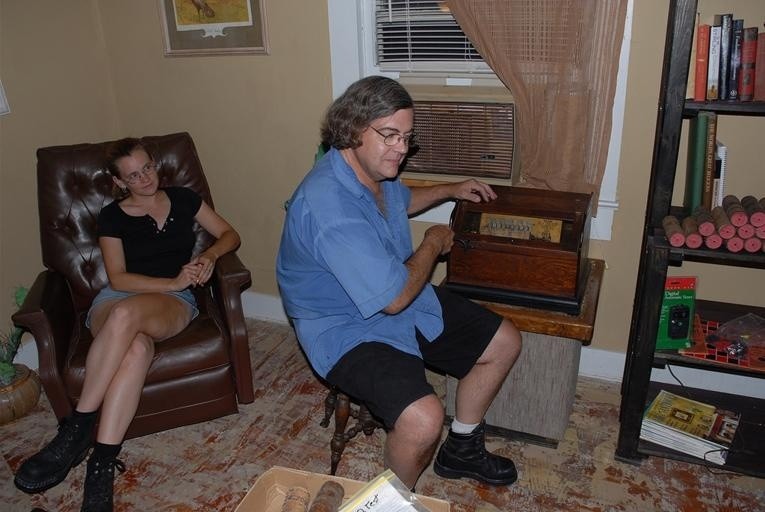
[318,393,377,478]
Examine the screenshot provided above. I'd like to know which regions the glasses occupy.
[121,161,156,185]
[369,124,416,146]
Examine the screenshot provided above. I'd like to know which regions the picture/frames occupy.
[159,0,268,59]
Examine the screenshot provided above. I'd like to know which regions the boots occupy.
[80,449,126,512]
[14,411,97,494]
[434,425,518,486]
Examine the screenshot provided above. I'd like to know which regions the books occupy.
[694,13,765,102]
[639,389,742,466]
[684,114,728,216]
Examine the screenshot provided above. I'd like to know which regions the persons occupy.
[14,136,241,512]
[275,75,523,492]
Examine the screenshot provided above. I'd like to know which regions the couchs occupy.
[11,131,254,443]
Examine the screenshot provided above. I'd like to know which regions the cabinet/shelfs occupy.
[614,1,764,483]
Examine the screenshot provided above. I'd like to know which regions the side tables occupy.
[432,258,606,441]
